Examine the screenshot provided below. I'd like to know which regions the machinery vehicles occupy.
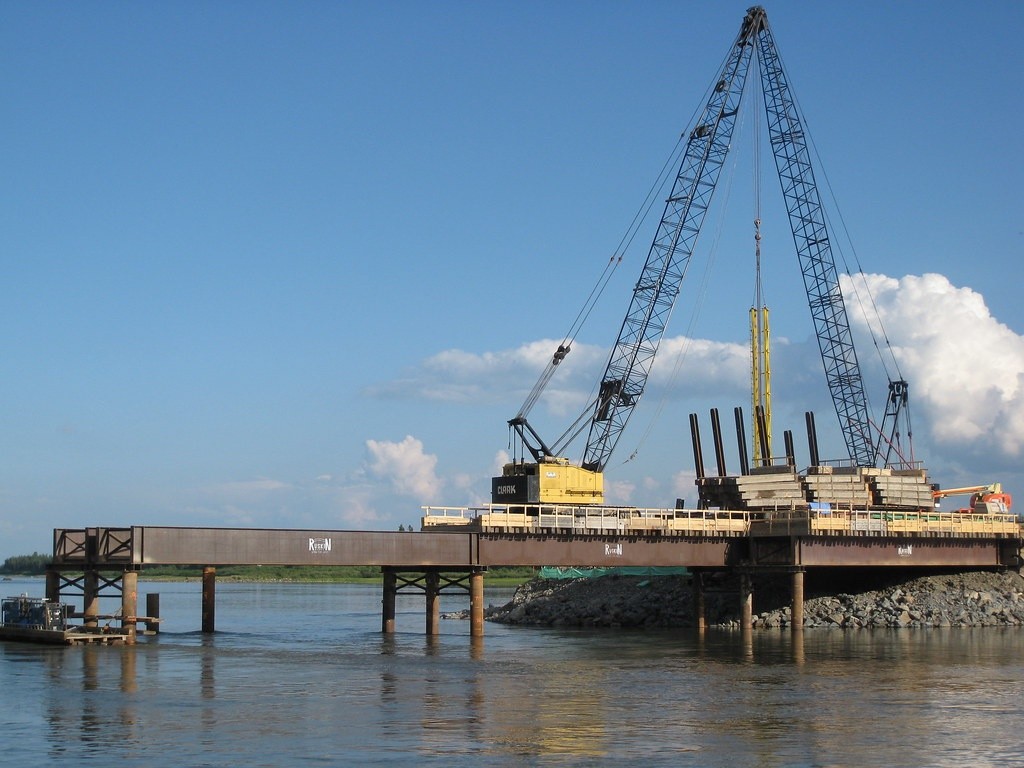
[934,483,1011,514]
[490,6,919,505]
